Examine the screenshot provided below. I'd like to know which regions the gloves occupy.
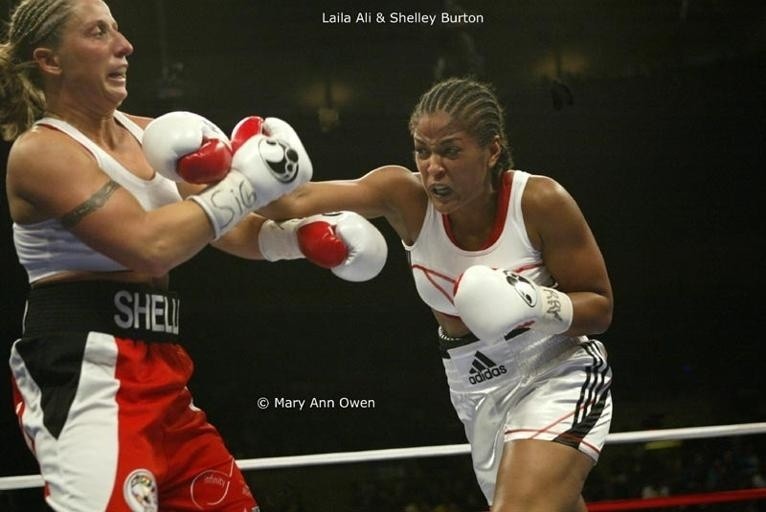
[143,111,231,186]
[453,265,573,344]
[260,210,388,281]
[186,115,314,241]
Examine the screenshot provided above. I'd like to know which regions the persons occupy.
[0,0,389,512]
[138,73,620,511]
